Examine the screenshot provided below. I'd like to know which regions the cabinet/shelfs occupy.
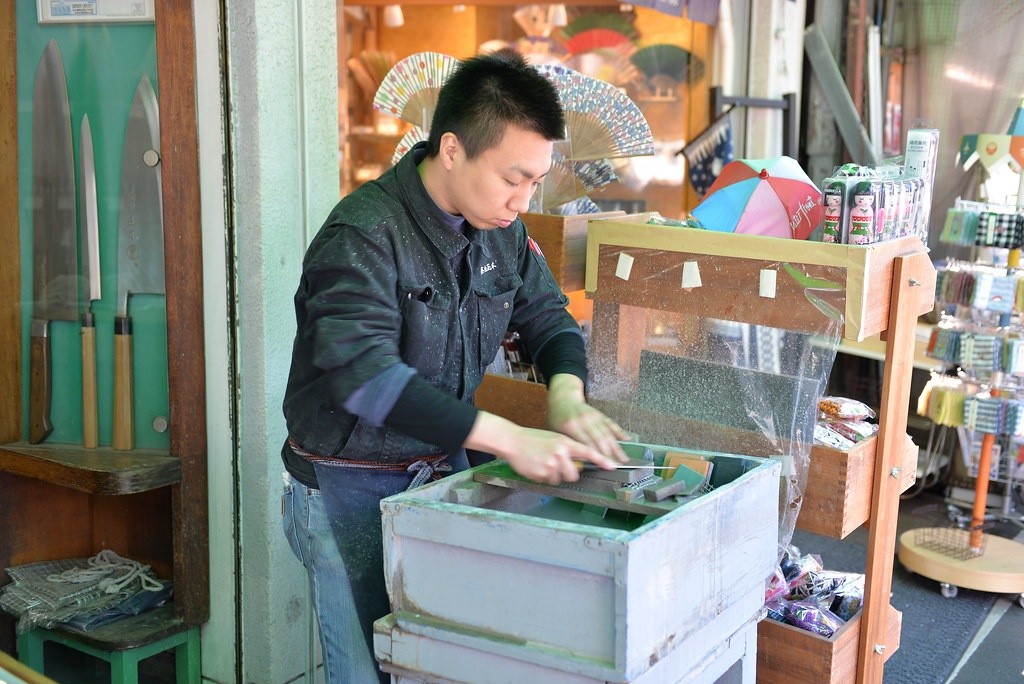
[473,209,937,684]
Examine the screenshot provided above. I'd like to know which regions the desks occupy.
[806,337,960,501]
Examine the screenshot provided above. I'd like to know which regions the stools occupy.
[15,598,202,684]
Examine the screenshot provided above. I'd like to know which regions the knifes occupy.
[573,459,678,477]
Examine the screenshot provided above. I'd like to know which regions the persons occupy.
[279,55,631,684]
